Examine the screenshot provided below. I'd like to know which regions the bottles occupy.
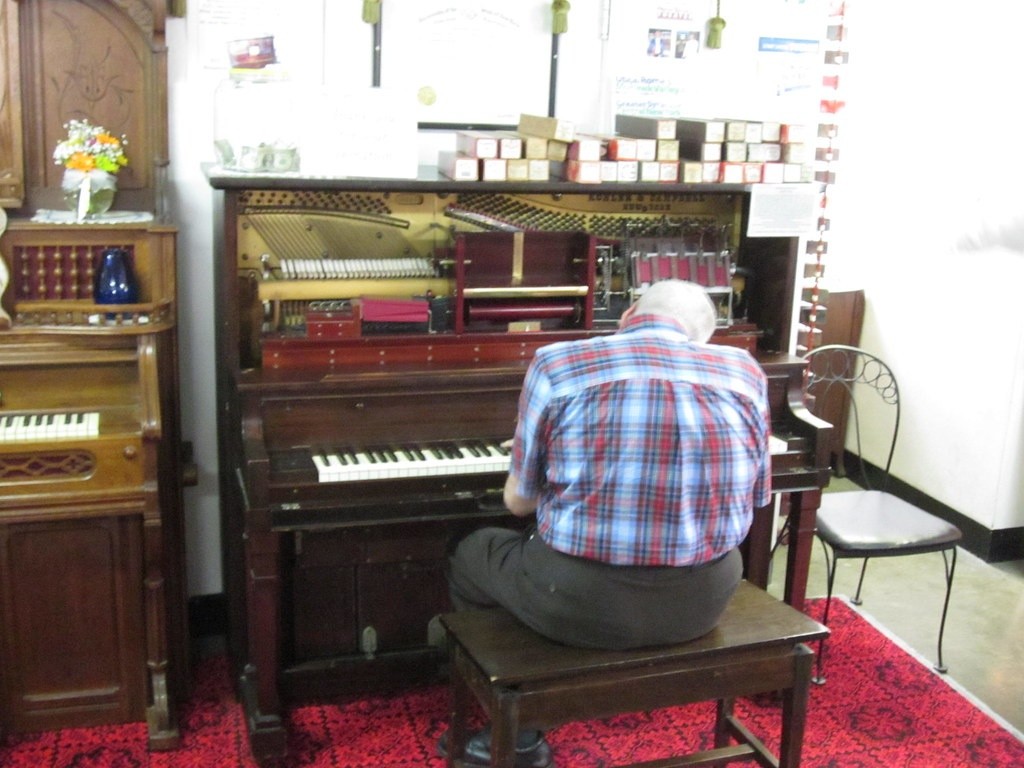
[93,248,138,324]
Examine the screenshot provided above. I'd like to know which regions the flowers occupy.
[49,115,129,175]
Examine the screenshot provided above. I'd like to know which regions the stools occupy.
[445,572,830,768]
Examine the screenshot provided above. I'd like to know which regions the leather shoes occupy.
[437,729,554,768]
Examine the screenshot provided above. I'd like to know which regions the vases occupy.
[58,164,116,219]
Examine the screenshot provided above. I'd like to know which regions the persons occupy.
[421,278,773,768]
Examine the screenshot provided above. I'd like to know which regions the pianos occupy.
[234,344,834,761]
[0,333,198,752]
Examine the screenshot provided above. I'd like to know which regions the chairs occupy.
[770,336,963,680]
